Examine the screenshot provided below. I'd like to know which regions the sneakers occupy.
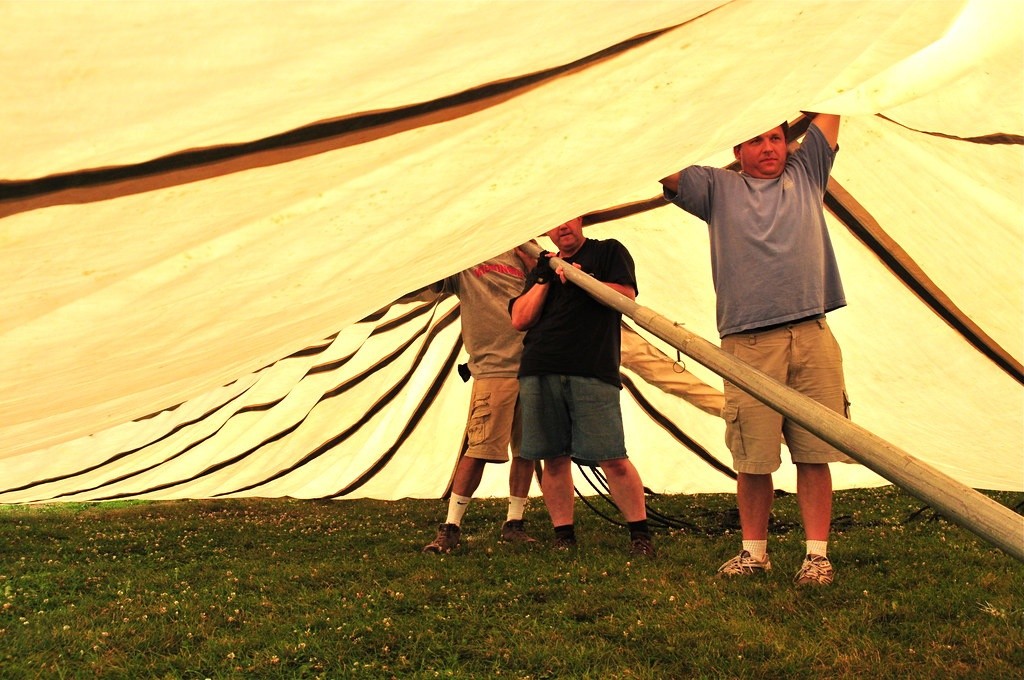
[423,522,462,555]
[628,539,654,562]
[792,554,834,589]
[715,550,772,579]
[500,519,536,543]
[552,539,578,551]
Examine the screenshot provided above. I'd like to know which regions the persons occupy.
[657,114,851,591]
[419,238,545,558]
[506,216,650,547]
